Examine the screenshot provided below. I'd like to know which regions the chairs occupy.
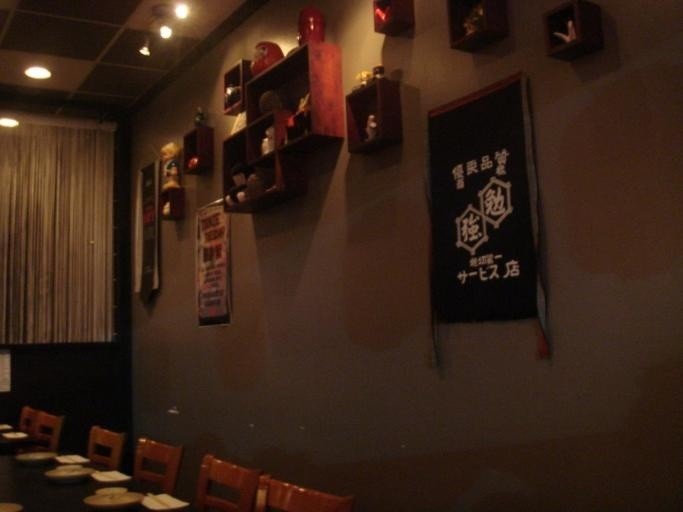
[18,406,42,433]
[194,453,261,512]
[133,436,184,495]
[85,425,127,471]
[256,475,354,512]
[33,411,65,451]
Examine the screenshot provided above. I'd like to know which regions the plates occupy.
[0,503,23,512]
[15,450,58,461]
[45,465,95,483]
[1,430,28,440]
[89,470,132,484]
[84,492,144,508]
[141,493,190,511]
[55,454,90,465]
[0,423,12,430]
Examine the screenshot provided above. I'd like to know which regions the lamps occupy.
[139,39,151,56]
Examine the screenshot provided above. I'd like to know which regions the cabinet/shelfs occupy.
[222,59,249,116]
[373,0,414,35]
[346,80,402,153]
[223,42,345,213]
[183,129,213,177]
[160,189,185,219]
[543,1,604,61]
[446,0,507,51]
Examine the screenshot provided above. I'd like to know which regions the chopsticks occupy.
[96,471,117,480]
[146,492,170,507]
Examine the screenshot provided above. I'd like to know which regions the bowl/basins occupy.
[95,487,127,495]
